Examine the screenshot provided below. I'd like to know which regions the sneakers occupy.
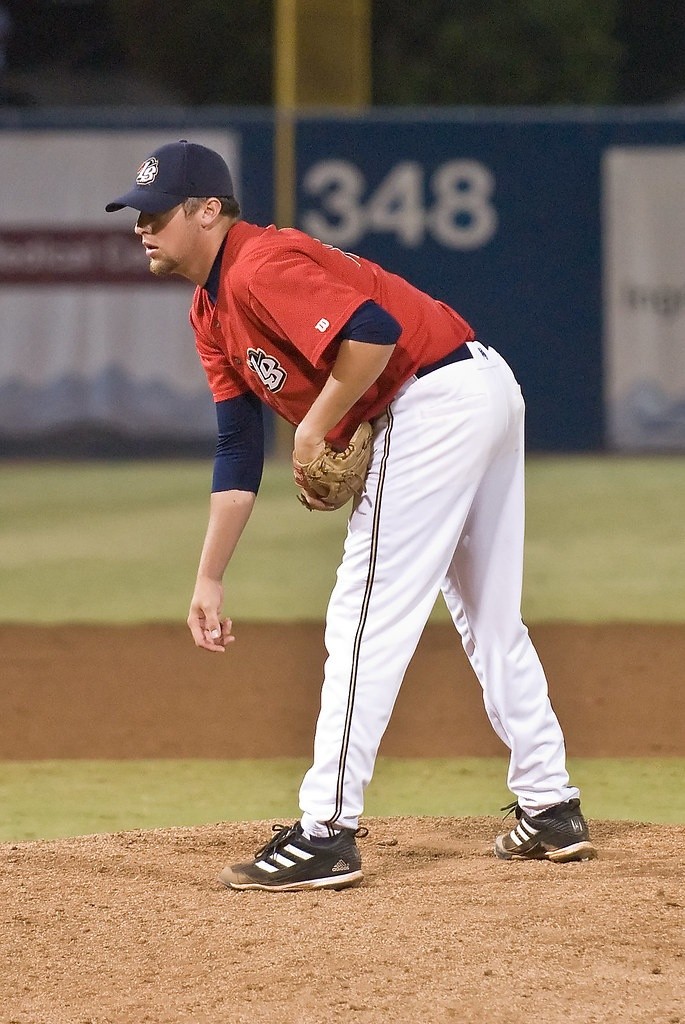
[217,821,369,892]
[493,800,598,862]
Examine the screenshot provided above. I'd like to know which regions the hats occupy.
[105,139,233,216]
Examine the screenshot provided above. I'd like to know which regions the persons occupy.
[105,138,595,893]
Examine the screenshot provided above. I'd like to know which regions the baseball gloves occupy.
[290,419,374,513]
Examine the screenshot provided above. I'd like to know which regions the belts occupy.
[414,337,501,379]
